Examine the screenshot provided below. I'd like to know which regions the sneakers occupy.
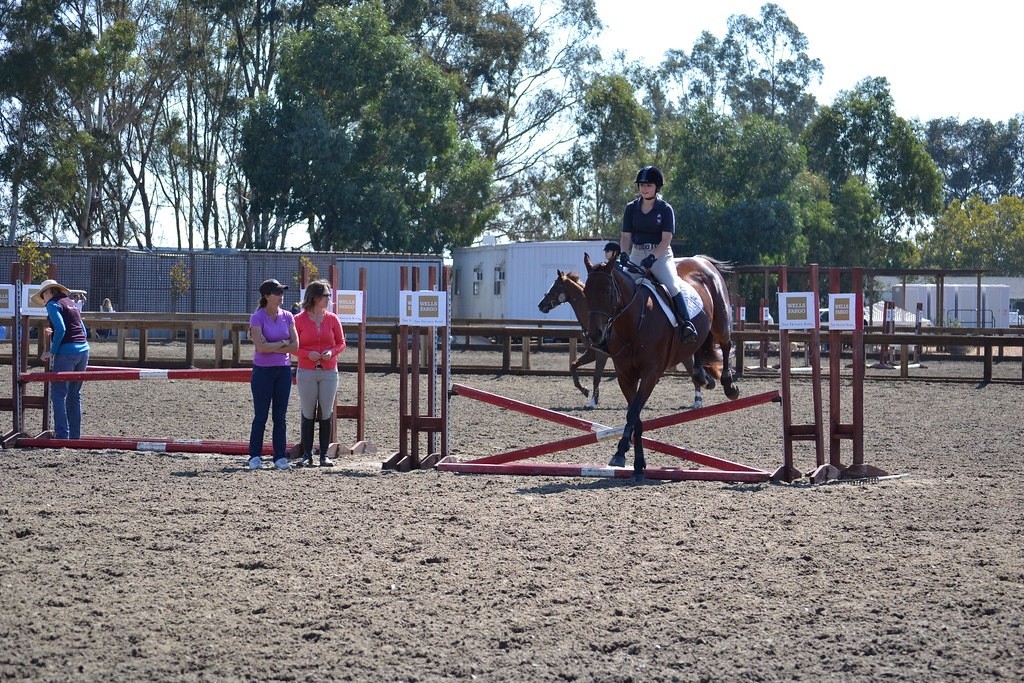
[249,456,262,469]
[275,458,289,469]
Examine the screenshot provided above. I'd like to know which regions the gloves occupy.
[620,252,629,267]
[641,254,657,269]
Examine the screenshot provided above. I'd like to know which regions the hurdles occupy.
[0,261,889,483]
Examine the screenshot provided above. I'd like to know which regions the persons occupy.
[589,165,699,356]
[291,278,347,468]
[601,242,621,271]
[90,298,117,341]
[247,278,300,470]
[29,280,90,439]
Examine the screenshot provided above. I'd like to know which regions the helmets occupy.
[603,242,621,251]
[634,166,663,186]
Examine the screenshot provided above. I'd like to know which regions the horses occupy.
[582,251,740,481]
[538,268,721,410]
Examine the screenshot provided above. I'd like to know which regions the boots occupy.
[297,413,315,466]
[672,292,694,344]
[319,415,333,467]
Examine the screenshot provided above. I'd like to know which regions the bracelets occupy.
[280,338,285,344]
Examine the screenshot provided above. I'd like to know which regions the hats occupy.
[259,279,289,294]
[31,279,71,306]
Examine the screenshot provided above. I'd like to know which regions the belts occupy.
[634,243,658,250]
[315,364,323,370]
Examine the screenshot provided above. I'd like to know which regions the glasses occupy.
[322,294,332,298]
[273,290,285,296]
